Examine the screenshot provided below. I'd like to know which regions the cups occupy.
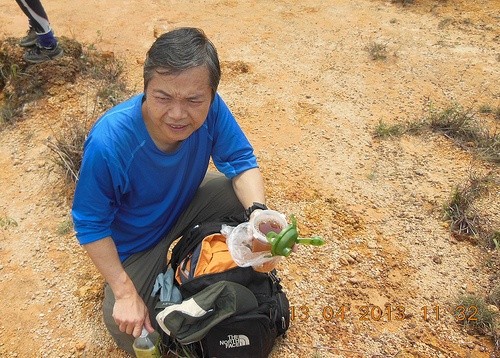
[249,209,288,272]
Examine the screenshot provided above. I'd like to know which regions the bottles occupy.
[132,325,165,358]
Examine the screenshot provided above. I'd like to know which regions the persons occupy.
[15,0,64,64]
[71,26,299,358]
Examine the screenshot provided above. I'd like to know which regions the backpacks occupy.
[152,217,289,358]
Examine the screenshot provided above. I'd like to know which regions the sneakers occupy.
[24,39,64,63]
[18,25,39,46]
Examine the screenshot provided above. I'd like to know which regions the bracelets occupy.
[244,201,268,221]
